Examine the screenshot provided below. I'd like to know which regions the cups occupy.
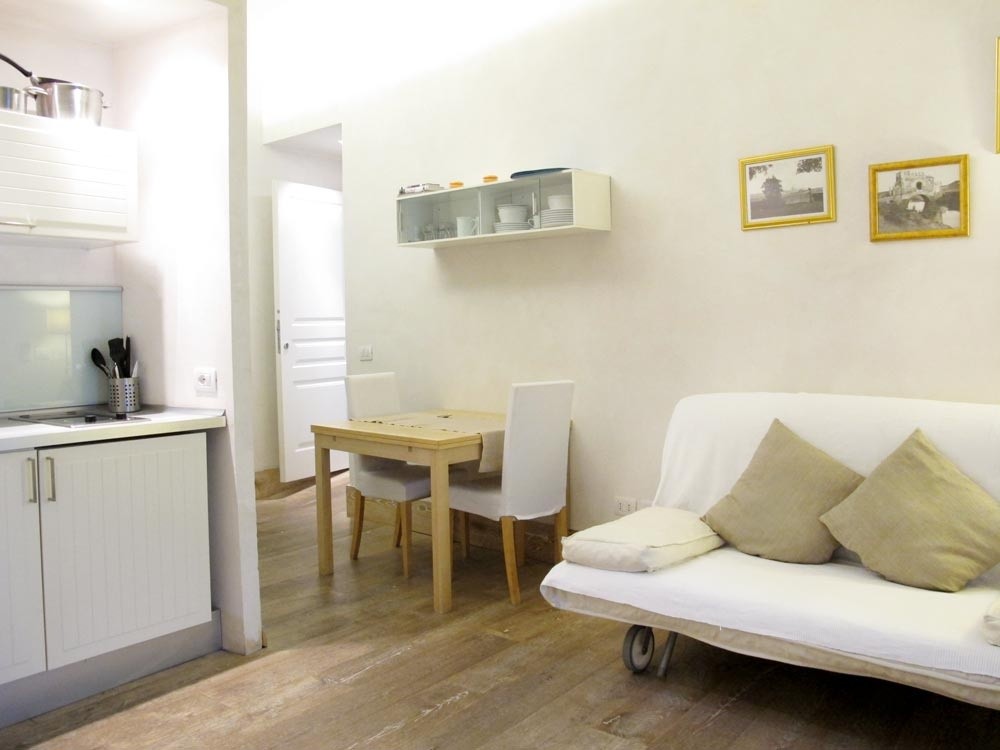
[456,217,478,237]
[423,223,456,240]
[527,215,541,229]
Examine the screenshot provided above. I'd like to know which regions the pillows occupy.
[817,428,1000,592]
[698,417,866,564]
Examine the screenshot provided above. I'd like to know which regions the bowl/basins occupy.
[547,194,572,209]
[496,204,529,223]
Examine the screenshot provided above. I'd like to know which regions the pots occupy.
[0,53,113,126]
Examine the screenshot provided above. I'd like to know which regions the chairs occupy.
[345,371,468,581]
[451,381,575,604]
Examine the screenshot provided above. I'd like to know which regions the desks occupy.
[311,409,506,614]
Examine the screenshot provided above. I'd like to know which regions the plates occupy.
[493,221,529,233]
[539,208,573,228]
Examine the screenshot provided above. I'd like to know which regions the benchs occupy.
[541,392,1000,712]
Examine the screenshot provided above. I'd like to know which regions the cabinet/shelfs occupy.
[0,108,138,248]
[0,431,211,685]
[395,170,611,250]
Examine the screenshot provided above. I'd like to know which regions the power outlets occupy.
[615,496,637,516]
[193,366,218,393]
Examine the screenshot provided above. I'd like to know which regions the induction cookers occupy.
[8,409,151,428]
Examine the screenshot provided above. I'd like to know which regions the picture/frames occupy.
[869,154,971,244]
[738,145,837,230]
